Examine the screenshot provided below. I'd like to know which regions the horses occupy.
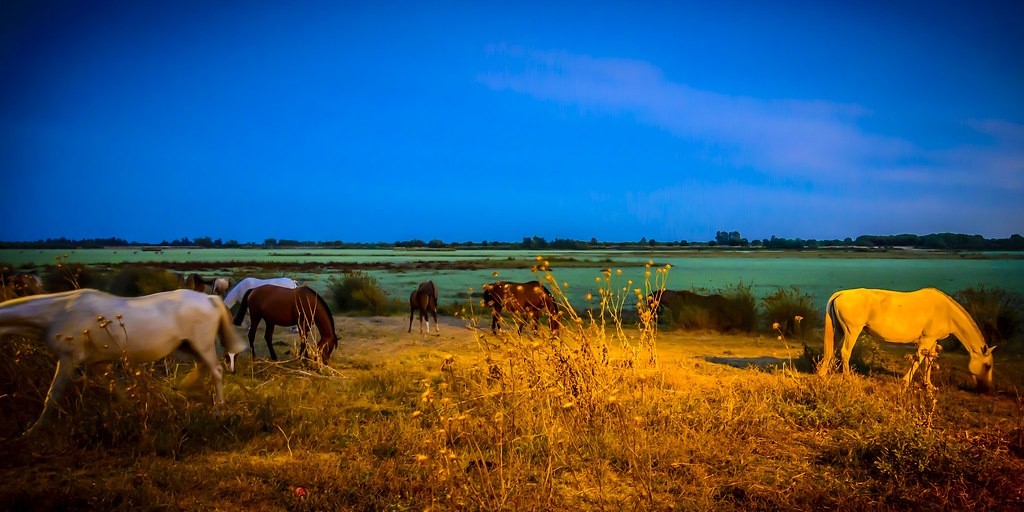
[483,280,561,339]
[407,280,440,338]
[0,261,341,366]
[0,289,247,440]
[815,287,997,394]
